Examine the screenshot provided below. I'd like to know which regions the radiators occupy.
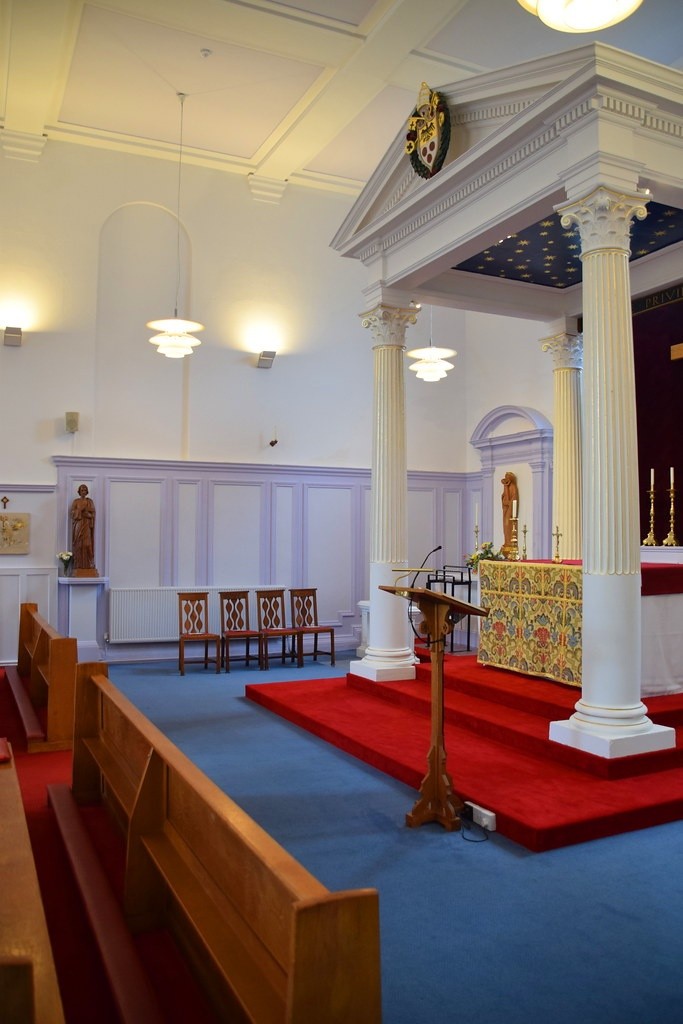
[104,585,288,644]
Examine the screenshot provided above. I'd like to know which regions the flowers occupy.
[56,551,73,573]
[461,541,519,575]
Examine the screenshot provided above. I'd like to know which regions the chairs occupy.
[254,590,300,670]
[288,588,335,668]
[217,591,264,673]
[177,592,221,676]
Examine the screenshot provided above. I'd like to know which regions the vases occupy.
[62,570,69,578]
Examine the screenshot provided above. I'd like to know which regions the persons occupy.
[70,484,96,569]
[501,473,519,547]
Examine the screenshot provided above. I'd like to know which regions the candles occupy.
[475,503,479,526]
[671,467,674,489]
[512,500,517,518]
[523,504,527,525]
[651,468,654,491]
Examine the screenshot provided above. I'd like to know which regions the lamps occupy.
[145,90,204,358]
[516,0,643,33]
[637,188,650,195]
[65,411,80,434]
[406,306,457,382]
[256,351,276,369]
[3,326,22,346]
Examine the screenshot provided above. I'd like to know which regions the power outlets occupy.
[465,801,496,831]
